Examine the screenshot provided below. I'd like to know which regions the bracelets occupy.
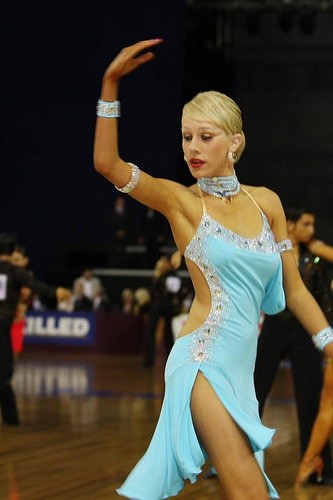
[310,325,333,352]
[272,238,297,256]
[114,162,140,194]
[96,98,122,119]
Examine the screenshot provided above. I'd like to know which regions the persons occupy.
[293,238,333,486]
[11,195,195,376]
[203,206,333,487]
[94,38,333,500]
[0,241,57,429]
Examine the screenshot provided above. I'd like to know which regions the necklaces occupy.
[197,168,242,205]
